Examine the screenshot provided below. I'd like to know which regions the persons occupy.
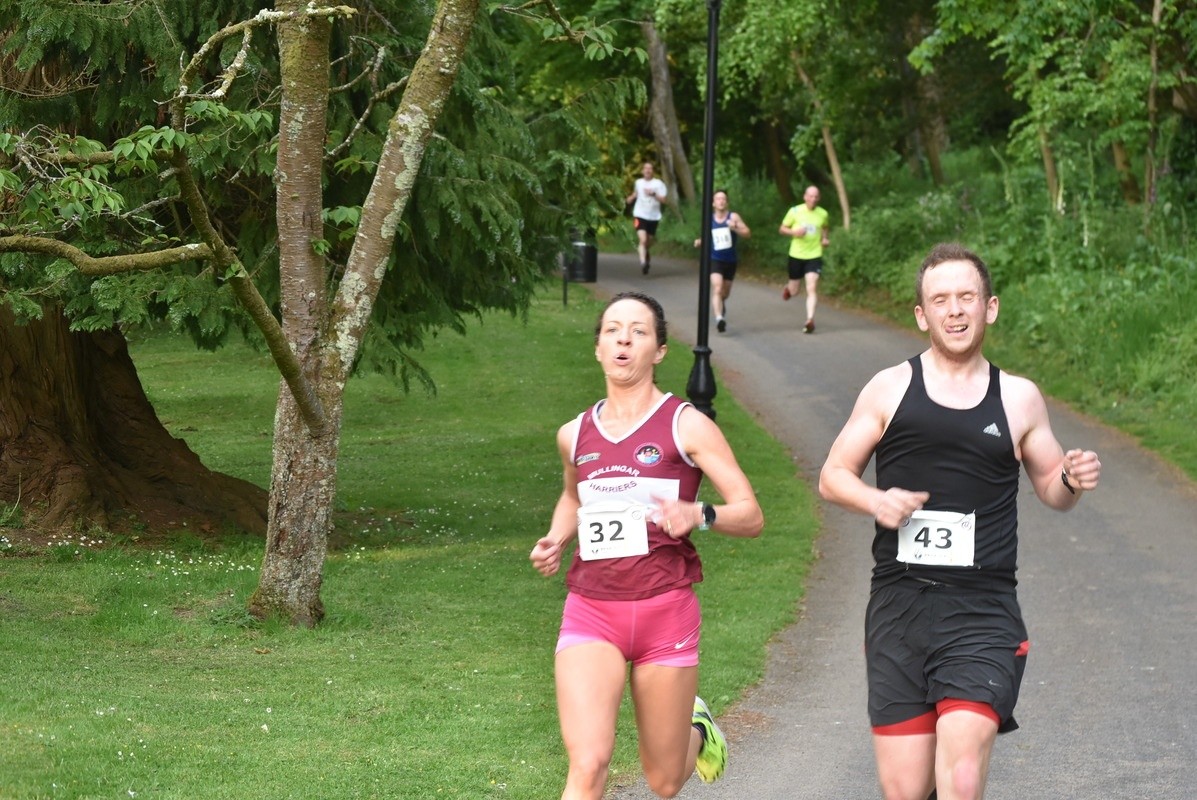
[527,291,765,799]
[778,184,831,333]
[817,242,1101,800]
[692,188,750,333]
[625,162,669,275]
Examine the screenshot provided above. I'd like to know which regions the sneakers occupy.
[691,695,728,785]
[783,288,792,300]
[804,321,815,335]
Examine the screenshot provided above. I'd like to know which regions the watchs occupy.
[696,501,719,534]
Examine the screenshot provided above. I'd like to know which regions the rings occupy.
[667,520,672,530]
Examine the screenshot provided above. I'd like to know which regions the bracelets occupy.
[1060,467,1077,496]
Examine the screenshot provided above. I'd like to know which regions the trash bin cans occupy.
[563,226,598,283]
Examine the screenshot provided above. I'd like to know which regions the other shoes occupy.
[716,319,727,334]
[643,262,650,275]
